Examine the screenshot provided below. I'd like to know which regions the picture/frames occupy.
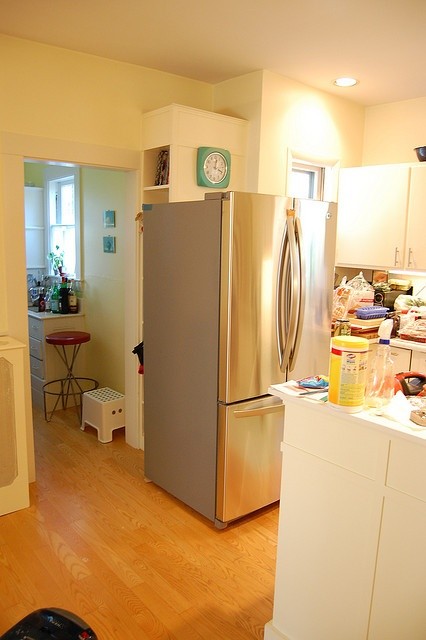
[103,210,116,228]
[102,235,115,252]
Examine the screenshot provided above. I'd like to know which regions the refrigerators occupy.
[144,191,338,529]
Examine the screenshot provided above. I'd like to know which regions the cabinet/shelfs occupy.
[333,162,425,272]
[389,338,426,377]
[1,334,31,518]
[25,187,45,285]
[28,311,87,412]
[263,382,426,640]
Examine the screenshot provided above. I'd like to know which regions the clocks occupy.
[196,148,231,189]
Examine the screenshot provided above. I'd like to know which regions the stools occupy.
[41,330,99,424]
[80,387,126,443]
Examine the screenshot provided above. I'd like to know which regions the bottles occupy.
[68,279,78,314]
[45,289,51,311]
[68,280,71,291]
[38,288,45,312]
[366,340,396,407]
[59,277,68,314]
[65,278,67,284]
[50,280,59,314]
[41,275,44,287]
[34,281,41,306]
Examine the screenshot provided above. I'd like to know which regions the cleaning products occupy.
[366,315,400,408]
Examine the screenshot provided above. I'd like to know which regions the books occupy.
[266,379,329,407]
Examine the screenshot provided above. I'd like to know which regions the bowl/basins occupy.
[413,145,426,162]
